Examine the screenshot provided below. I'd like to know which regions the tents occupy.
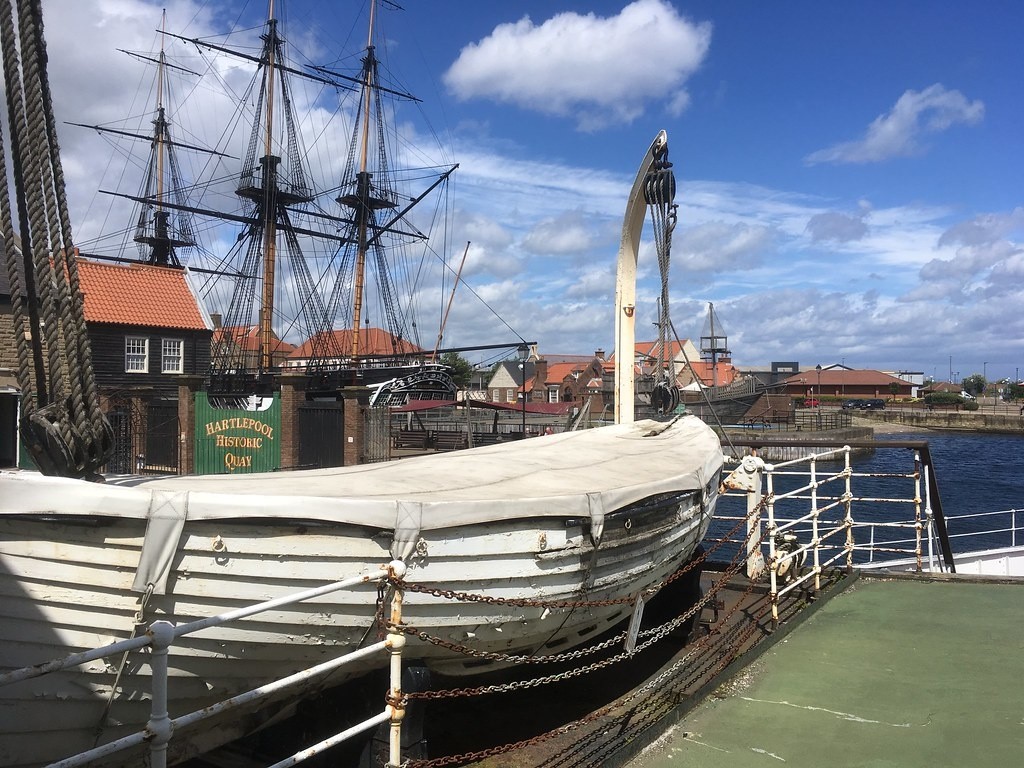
[679,382,709,401]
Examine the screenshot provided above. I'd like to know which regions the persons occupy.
[546,427,553,435]
[1020,399,1024,415]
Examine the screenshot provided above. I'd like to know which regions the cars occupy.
[842,399,863,410]
[859,398,885,410]
[802,398,819,408]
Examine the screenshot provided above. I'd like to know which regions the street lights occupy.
[815,364,822,428]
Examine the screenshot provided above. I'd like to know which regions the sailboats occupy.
[0,3,876,768]
